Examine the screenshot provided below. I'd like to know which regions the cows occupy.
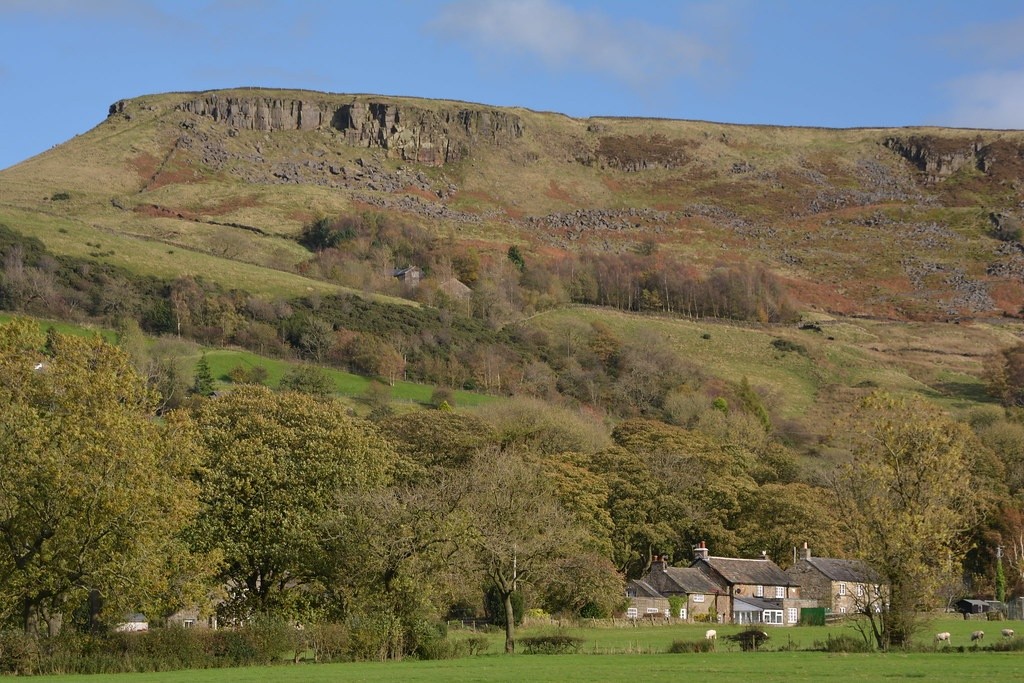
[705,630,717,639]
[934,632,951,645]
[971,631,984,644]
[1001,628,1014,639]
